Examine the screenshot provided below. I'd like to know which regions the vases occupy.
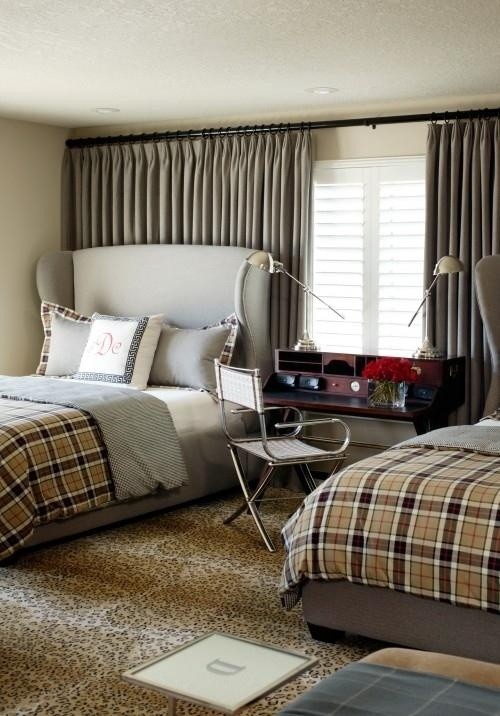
[367,380,405,408]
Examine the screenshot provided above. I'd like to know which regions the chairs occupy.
[213,358,351,551]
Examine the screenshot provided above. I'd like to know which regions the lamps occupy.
[246,250,345,352]
[407,255,464,358]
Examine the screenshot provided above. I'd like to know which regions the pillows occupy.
[149,313,239,398]
[35,300,92,376]
[77,312,166,391]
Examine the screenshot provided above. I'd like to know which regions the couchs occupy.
[275,647,500,715]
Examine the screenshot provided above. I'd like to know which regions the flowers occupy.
[362,358,418,407]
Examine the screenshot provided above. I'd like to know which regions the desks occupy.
[247,348,465,514]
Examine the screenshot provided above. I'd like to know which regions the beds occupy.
[0,243,274,564]
[280,255,499,667]
